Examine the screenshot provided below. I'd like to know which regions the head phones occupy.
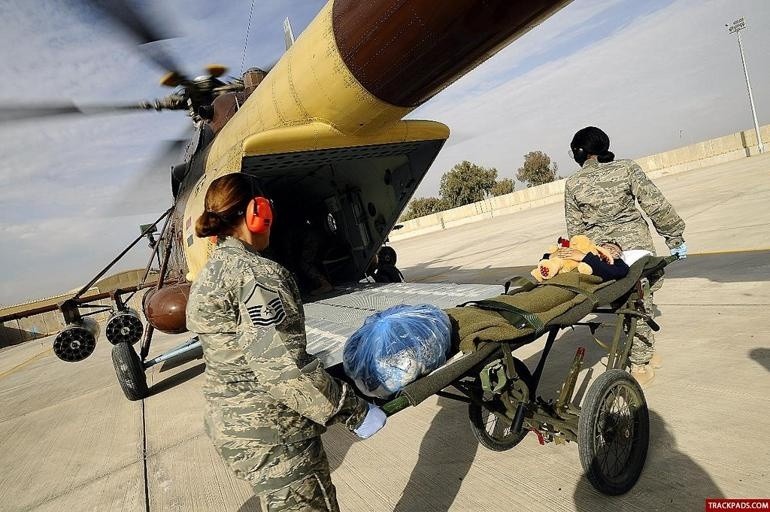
[573,141,588,163]
[208,171,274,243]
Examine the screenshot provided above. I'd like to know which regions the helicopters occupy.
[1,1,574,401]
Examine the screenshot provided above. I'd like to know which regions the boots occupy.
[627,361,657,390]
[647,352,663,371]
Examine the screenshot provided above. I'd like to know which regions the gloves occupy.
[351,405,388,440]
[669,240,688,260]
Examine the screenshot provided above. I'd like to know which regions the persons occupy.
[289,224,335,297]
[564,124,690,390]
[531,235,614,284]
[184,172,389,512]
[541,238,630,284]
[358,246,401,283]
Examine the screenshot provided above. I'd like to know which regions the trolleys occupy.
[316,252,688,496]
